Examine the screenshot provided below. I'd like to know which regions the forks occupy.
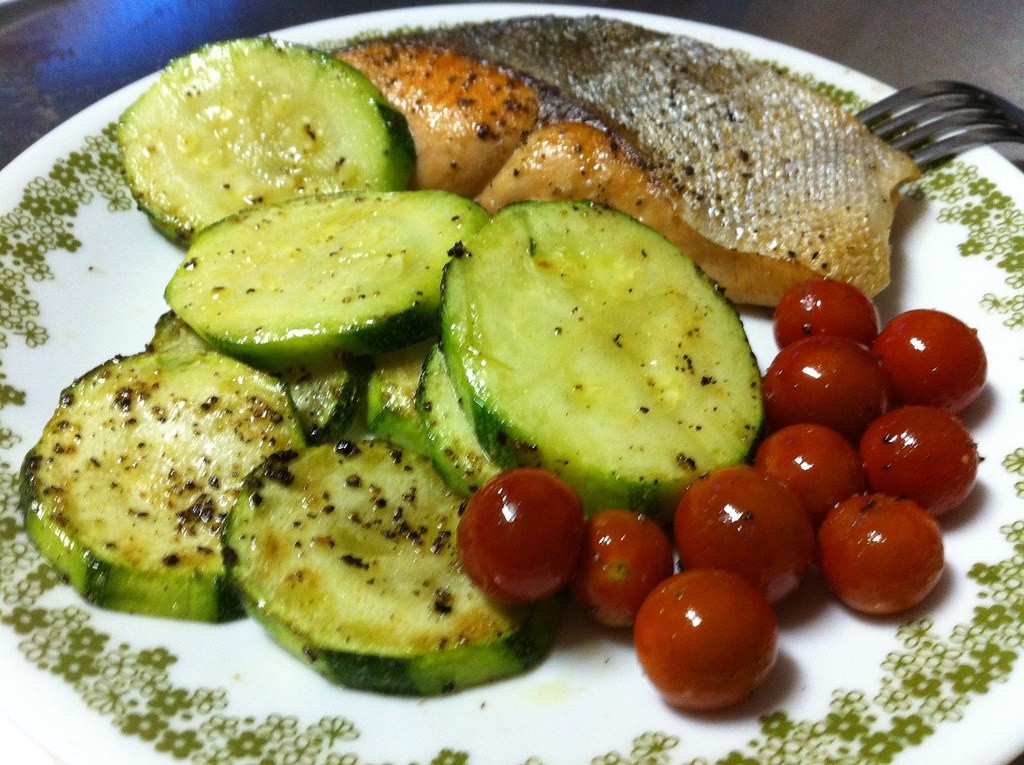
[854,80,1024,166]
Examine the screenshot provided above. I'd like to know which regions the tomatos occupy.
[456,276,988,713]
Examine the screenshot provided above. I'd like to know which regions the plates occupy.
[0,3,1024,765]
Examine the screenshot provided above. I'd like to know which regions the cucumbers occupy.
[18,37,766,701]
